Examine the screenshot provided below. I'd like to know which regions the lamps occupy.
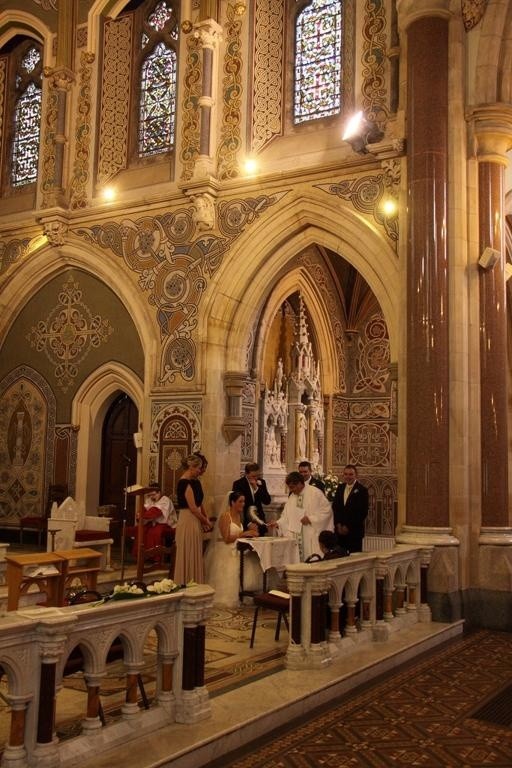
[342,109,385,154]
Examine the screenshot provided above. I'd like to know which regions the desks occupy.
[237,536,298,602]
[4,547,104,611]
[21,515,45,552]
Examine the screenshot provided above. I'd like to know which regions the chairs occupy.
[247,570,293,648]
[45,495,113,569]
[305,553,323,562]
[65,589,147,724]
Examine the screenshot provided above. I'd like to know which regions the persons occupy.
[126,483,177,560]
[173,453,369,608]
